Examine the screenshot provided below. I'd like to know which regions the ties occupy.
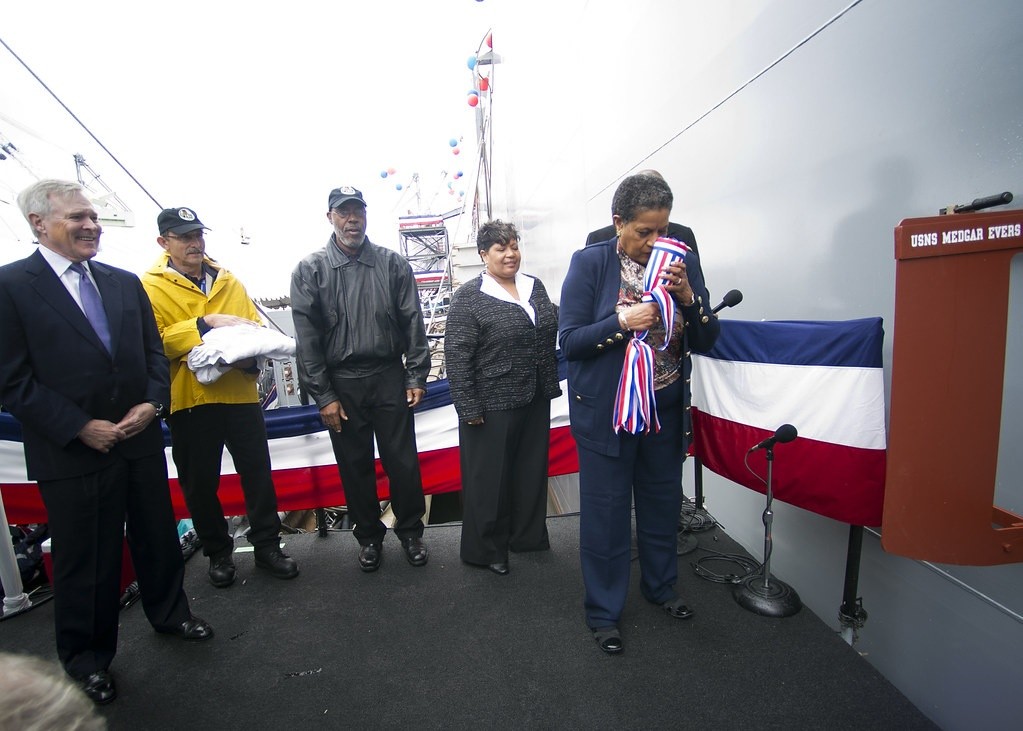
[69,262,111,354]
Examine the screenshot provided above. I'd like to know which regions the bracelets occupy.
[618,311,630,332]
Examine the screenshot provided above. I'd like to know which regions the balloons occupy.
[395,184,401,190]
[449,180,464,202]
[450,138,457,147]
[467,55,476,70]
[454,170,464,179]
[486,33,492,48]
[466,89,479,107]
[452,147,460,155]
[380,167,397,178]
[478,77,488,90]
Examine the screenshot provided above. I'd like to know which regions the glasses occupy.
[160,232,207,241]
[330,208,367,218]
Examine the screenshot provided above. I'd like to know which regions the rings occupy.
[675,278,681,285]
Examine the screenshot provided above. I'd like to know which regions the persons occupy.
[291,185,433,572]
[141,205,298,586]
[0,650,109,731]
[0,179,213,704]
[445,220,563,575]
[557,169,720,651]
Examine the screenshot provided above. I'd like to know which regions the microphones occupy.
[750,423,798,451]
[711,289,744,314]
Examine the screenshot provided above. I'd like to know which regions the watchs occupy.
[150,400,165,417]
[690,290,696,304]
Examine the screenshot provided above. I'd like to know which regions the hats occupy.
[158,207,212,234]
[328,186,367,211]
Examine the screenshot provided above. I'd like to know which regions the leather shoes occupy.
[157,611,214,641]
[77,670,116,704]
[400,536,428,564]
[489,563,508,573]
[592,625,623,651]
[646,595,694,617]
[358,542,383,570]
[208,553,237,586]
[254,546,299,578]
